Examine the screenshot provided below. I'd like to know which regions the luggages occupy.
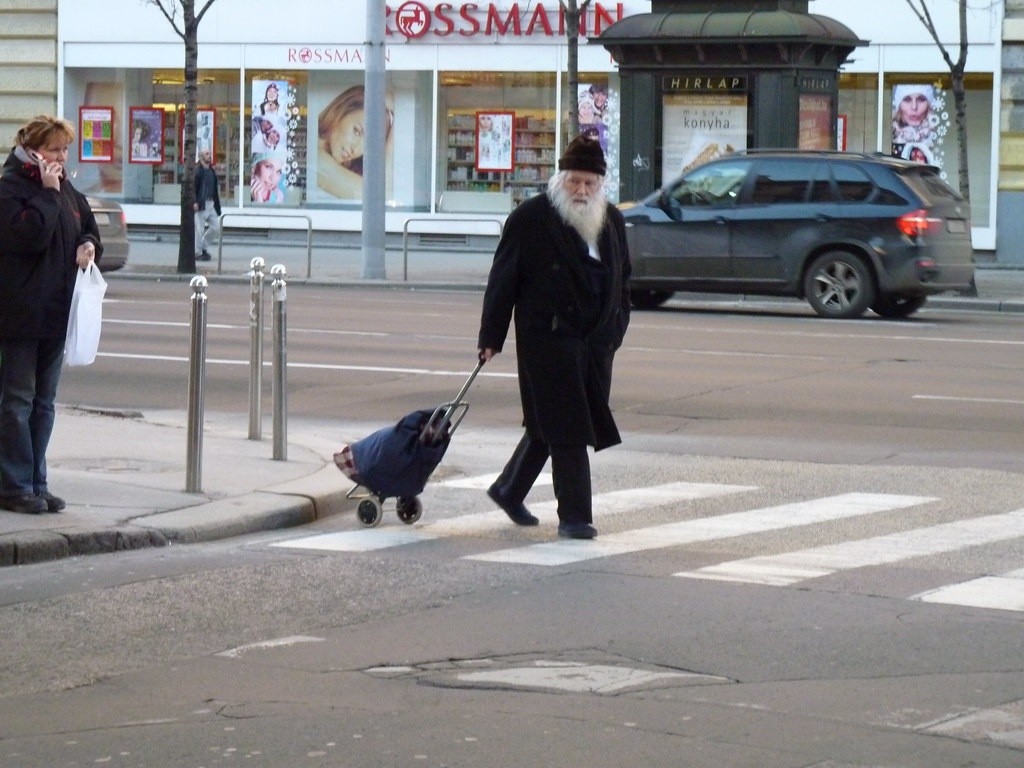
[333,348,487,527]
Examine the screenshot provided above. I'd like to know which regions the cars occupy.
[82,195,129,274]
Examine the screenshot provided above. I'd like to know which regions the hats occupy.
[895,84,934,113]
[264,83,280,102]
[270,125,285,142]
[262,114,278,128]
[558,134,607,176]
[251,150,286,175]
[578,90,601,115]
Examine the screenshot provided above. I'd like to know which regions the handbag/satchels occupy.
[62,261,107,368]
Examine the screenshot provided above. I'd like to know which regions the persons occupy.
[478,114,495,136]
[0,115,105,514]
[194,149,222,260]
[132,119,151,156]
[251,82,284,202]
[477,128,633,537]
[891,85,937,163]
[318,85,395,205]
[578,86,608,140]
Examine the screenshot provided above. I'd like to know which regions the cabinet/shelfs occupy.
[154,110,307,190]
[447,113,567,194]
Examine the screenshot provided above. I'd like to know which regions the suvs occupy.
[619,149,975,319]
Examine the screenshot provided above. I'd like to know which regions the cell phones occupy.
[29,149,50,170]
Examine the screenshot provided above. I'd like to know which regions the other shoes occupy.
[0,492,48,513]
[558,520,597,539]
[34,490,66,511]
[487,483,539,526]
[195,249,211,259]
